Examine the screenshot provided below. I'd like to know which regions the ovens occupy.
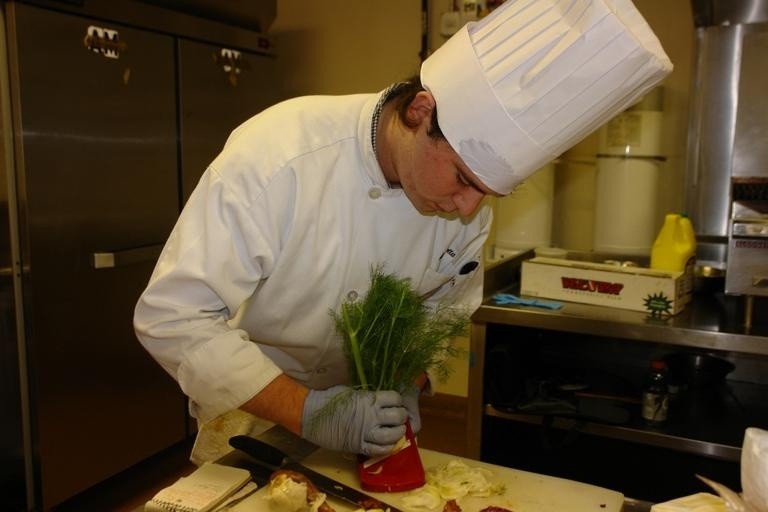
[0,1,274,511]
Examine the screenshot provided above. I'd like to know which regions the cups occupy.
[535,246,568,260]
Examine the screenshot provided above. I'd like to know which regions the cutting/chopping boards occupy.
[224,447,589,512]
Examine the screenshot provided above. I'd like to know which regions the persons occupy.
[132,74,508,470]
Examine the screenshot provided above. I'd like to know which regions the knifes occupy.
[228,432,423,512]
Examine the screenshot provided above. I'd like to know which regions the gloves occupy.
[300,384,409,460]
[399,385,424,434]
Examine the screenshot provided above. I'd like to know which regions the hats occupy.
[418,0,674,196]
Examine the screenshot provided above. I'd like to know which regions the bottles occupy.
[640,359,670,426]
[650,209,696,273]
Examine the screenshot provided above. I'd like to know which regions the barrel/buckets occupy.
[649,210,698,308]
